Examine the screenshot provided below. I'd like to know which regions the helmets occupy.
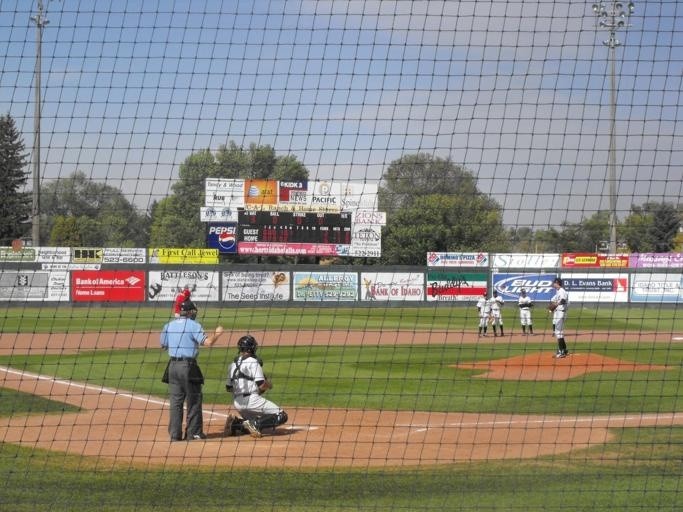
[179,301,198,320]
[237,335,258,354]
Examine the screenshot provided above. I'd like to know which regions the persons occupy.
[477,292,491,337]
[225,335,288,438]
[549,278,568,358]
[174,289,190,317]
[518,289,533,336]
[159,299,224,440]
[488,290,505,336]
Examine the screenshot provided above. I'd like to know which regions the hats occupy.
[553,278,561,284]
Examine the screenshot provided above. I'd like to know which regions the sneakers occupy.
[192,432,206,439]
[241,419,262,438]
[550,353,565,358]
[224,414,238,436]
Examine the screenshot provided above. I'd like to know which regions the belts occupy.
[170,357,195,362]
[243,393,250,397]
[555,310,565,312]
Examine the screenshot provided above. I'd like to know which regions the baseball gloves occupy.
[547,302,557,312]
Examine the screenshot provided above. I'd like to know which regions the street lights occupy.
[592,0,634,254]
[29,0,50,247]
